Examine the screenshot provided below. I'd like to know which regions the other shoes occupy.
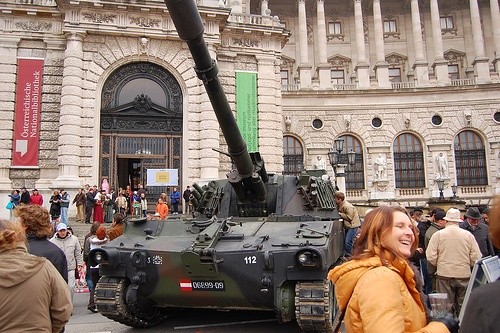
[176,212,179,214]
[88,306,99,313]
[85,221,91,224]
[173,212,176,214]
[340,256,350,262]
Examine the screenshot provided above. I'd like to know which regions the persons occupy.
[335,192,360,256]
[375,154,386,179]
[0,220,73,333]
[409,207,447,309]
[459,196,500,333]
[327,206,460,333]
[183,186,191,214]
[147,193,169,220]
[49,189,70,226]
[72,178,147,224]
[49,223,83,316]
[460,208,500,257]
[426,209,482,316]
[437,153,447,177]
[83,213,125,312]
[314,156,325,169]
[170,187,180,214]
[8,187,43,218]
[15,203,68,333]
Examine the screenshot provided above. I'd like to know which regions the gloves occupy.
[436,312,460,333]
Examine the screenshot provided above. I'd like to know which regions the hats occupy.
[32,189,38,191]
[20,187,27,189]
[465,207,482,219]
[57,222,67,231]
[443,208,464,223]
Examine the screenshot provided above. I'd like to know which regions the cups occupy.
[428,293,448,310]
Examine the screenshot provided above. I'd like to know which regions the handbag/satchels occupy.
[6,201,14,209]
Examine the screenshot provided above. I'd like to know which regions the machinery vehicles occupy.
[88,0,364,333]
